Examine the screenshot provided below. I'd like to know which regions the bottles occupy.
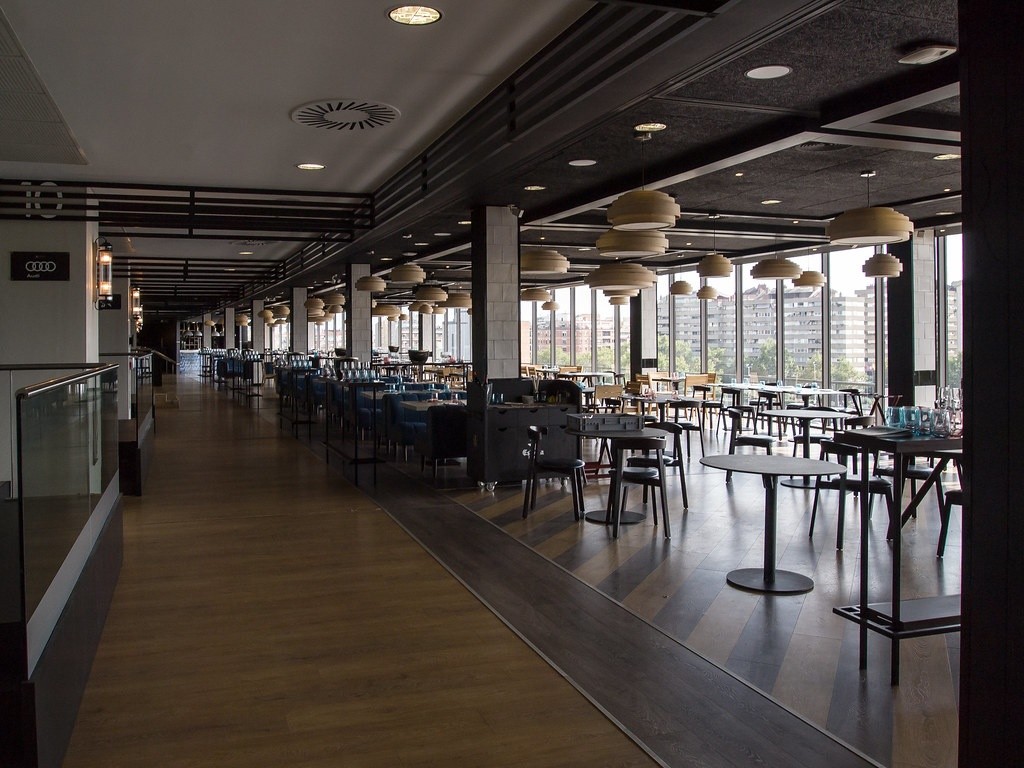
[797,383,801,393]
[777,380,783,391]
[811,381,817,393]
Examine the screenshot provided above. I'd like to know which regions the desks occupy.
[783,388,837,443]
[698,454,848,595]
[400,399,467,466]
[359,389,432,462]
[564,426,670,524]
[719,383,767,431]
[832,425,962,687]
[618,394,682,457]
[464,377,582,491]
[749,386,804,437]
[762,410,850,489]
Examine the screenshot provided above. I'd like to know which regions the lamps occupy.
[519,140,916,310]
[92,235,113,311]
[128,284,144,333]
[180,265,472,339]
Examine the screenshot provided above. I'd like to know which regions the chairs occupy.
[197,346,963,595]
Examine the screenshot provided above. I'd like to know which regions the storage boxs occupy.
[566,412,645,432]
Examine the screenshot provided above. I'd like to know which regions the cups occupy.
[885,407,905,429]
[398,385,406,393]
[904,407,921,436]
[444,384,449,392]
[730,378,736,386]
[951,388,962,410]
[743,378,749,386]
[760,381,765,386]
[671,390,678,400]
[397,377,403,384]
[932,410,950,438]
[937,387,951,409]
[919,407,932,435]
[948,410,963,437]
[431,393,438,401]
[429,384,434,390]
[451,394,458,403]
[389,384,395,394]
